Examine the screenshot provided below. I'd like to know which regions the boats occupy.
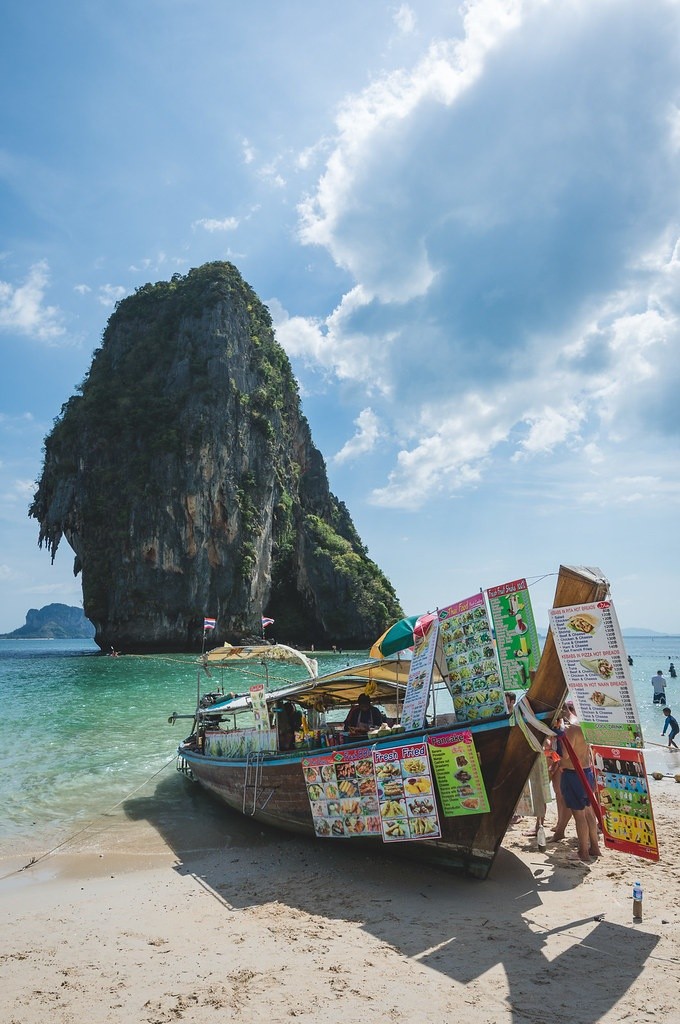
[177,561,610,878]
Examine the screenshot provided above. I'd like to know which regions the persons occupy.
[332,643,342,654]
[660,708,679,749]
[311,644,314,653]
[344,692,384,733]
[651,670,667,706]
[627,655,633,665]
[504,691,605,862]
[669,663,677,677]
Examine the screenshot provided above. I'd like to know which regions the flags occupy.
[261,615,275,628]
[203,617,216,630]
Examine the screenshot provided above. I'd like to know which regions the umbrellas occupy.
[368,613,438,661]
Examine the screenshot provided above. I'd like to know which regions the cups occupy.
[520,637,527,654]
[516,614,526,631]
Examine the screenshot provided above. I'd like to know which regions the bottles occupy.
[633,882,642,917]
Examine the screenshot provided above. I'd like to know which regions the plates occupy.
[452,747,465,754]
[454,770,471,784]
[460,798,479,810]
[514,649,531,658]
[514,623,528,634]
[305,760,438,840]
[457,787,475,798]
[439,606,506,722]
[456,755,470,769]
